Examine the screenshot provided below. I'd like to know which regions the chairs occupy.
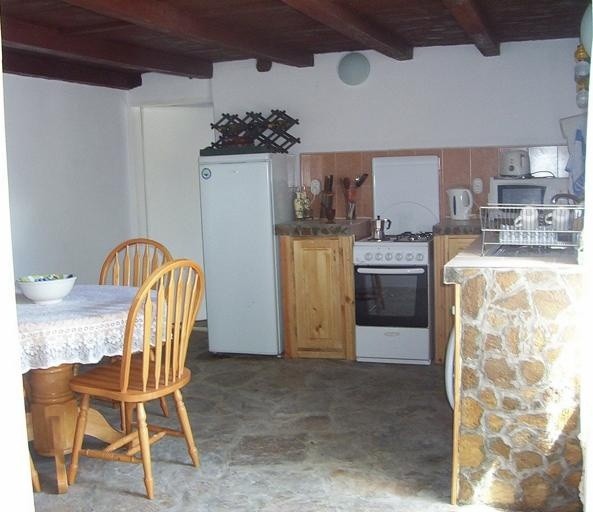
[64,258,206,500]
[71,237,176,452]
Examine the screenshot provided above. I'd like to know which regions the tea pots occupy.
[370,215,392,239]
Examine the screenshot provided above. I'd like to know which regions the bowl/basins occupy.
[19,273,75,305]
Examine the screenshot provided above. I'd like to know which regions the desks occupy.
[14,284,172,495]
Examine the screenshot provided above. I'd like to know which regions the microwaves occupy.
[488,178,573,222]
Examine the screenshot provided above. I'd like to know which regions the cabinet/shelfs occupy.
[432,216,487,365]
[274,218,372,361]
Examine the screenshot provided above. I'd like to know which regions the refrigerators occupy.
[197,152,296,358]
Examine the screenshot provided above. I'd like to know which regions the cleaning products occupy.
[568,129,587,198]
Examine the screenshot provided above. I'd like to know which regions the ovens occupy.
[355,267,433,365]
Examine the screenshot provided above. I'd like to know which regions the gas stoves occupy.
[353,228,434,266]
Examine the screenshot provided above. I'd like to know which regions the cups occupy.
[545,207,572,230]
[326,209,337,223]
[513,207,540,229]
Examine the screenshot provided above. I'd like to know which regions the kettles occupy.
[446,188,475,220]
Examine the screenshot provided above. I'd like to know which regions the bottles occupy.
[294,184,314,220]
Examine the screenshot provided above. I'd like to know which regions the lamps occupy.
[338,52,370,85]
